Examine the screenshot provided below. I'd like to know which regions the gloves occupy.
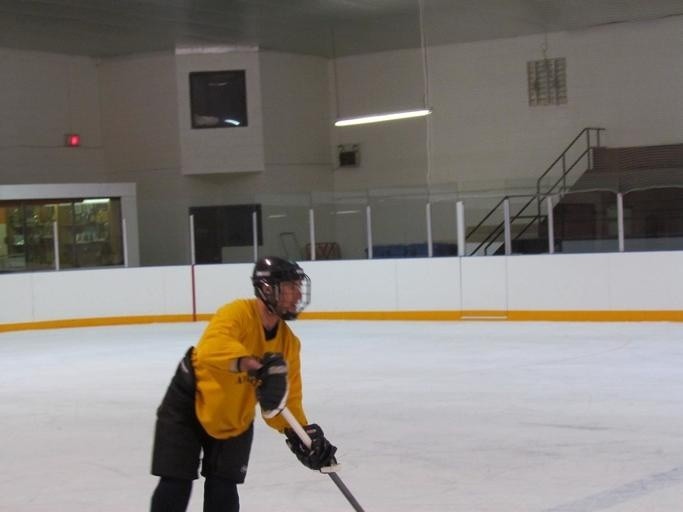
[284,424,338,473]
[248,351,288,420]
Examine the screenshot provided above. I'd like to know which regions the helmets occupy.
[253,257,304,284]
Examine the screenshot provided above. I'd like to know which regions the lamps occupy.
[330,16,432,127]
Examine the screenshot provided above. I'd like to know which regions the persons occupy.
[150,254,334,511]
